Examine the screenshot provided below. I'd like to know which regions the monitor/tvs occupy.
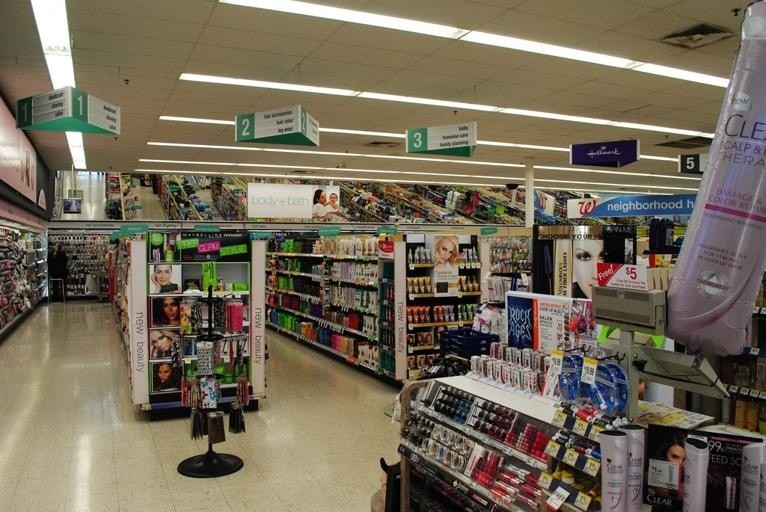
[63,199,82,214]
[68,190,83,200]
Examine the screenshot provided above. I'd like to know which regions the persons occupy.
[48,241,68,301]
[150,329,178,358]
[151,265,178,293]
[435,235,458,273]
[312,189,338,218]
[157,363,181,391]
[573,239,604,300]
[161,298,180,326]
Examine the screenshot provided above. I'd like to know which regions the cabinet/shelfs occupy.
[377,294,765,511]
[108,227,269,414]
[42,229,112,299]
[0,223,47,338]
[100,172,605,227]
[262,227,640,391]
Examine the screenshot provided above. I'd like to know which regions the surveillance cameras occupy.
[505,184,519,190]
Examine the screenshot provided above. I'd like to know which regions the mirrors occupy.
[52,168,654,227]
